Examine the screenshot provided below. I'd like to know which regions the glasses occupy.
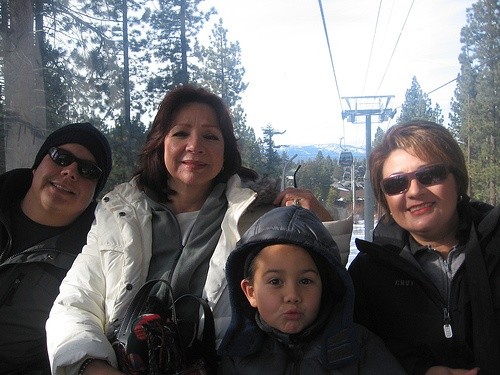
[49,147,103,179]
[380,164,453,196]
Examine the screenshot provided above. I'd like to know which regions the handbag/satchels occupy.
[106,279,216,375]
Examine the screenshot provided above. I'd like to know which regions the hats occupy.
[32,122,112,199]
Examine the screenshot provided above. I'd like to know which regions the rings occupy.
[293,199,299,206]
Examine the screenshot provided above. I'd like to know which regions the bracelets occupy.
[78,357,93,375]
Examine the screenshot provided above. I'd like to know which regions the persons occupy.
[209,206,407,375]
[348,120,500,375]
[44,86,353,375]
[0,122,112,375]
